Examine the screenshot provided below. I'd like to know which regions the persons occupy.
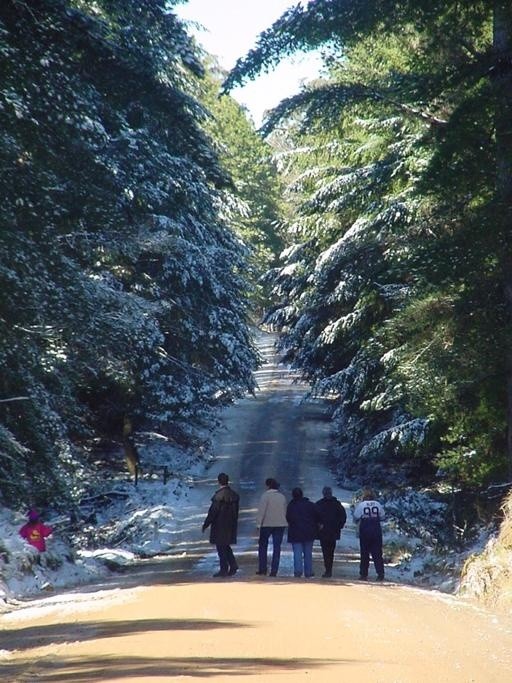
[19,510,53,551]
[286,488,324,578]
[256,479,289,576]
[316,487,346,577]
[353,491,386,580]
[202,473,239,577]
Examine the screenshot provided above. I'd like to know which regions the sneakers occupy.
[255,570,333,578]
[213,570,227,577]
[359,576,367,581]
[228,567,239,575]
[376,575,383,581]
